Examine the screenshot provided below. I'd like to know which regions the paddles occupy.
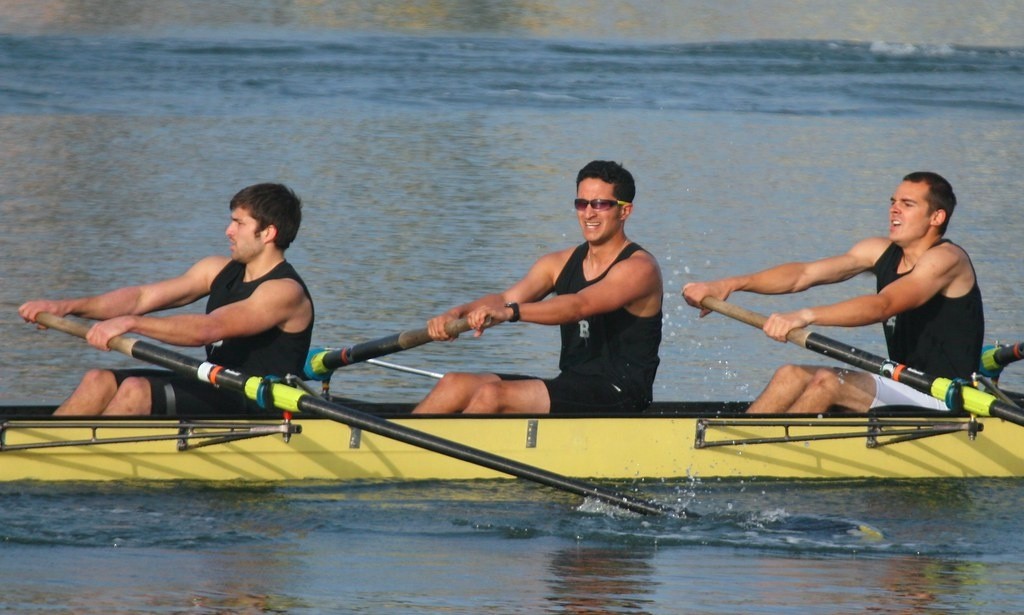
[978,340,1024,377]
[298,315,493,380]
[34,310,884,546]
[680,280,1024,427]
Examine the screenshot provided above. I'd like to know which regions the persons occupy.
[683,172,984,414]
[411,161,663,415]
[16,183,315,416]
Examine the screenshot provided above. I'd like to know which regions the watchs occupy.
[505,302,520,322]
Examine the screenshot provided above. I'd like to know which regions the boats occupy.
[1,402,1024,489]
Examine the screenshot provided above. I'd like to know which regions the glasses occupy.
[574,198,631,210]
